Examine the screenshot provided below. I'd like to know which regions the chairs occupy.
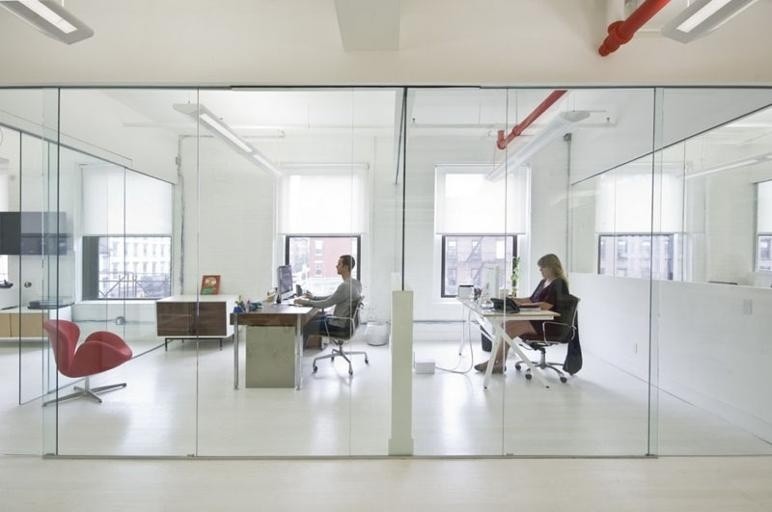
[313,295,368,375]
[42,319,134,406]
[515,294,581,384]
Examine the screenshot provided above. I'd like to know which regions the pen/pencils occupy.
[234,295,243,305]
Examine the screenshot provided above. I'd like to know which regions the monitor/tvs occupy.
[273,265,293,304]
[478,263,499,308]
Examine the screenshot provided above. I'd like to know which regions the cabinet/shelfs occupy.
[0,307,71,346]
[156,296,238,351]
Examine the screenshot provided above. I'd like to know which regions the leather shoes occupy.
[475,361,506,373]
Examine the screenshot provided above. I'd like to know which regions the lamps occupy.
[481,88,589,183]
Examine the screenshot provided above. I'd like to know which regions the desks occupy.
[455,296,554,390]
[230,297,320,387]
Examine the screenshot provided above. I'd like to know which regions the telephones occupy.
[490,297,520,313]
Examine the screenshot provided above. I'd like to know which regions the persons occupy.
[290,255,362,348]
[474,253,570,372]
